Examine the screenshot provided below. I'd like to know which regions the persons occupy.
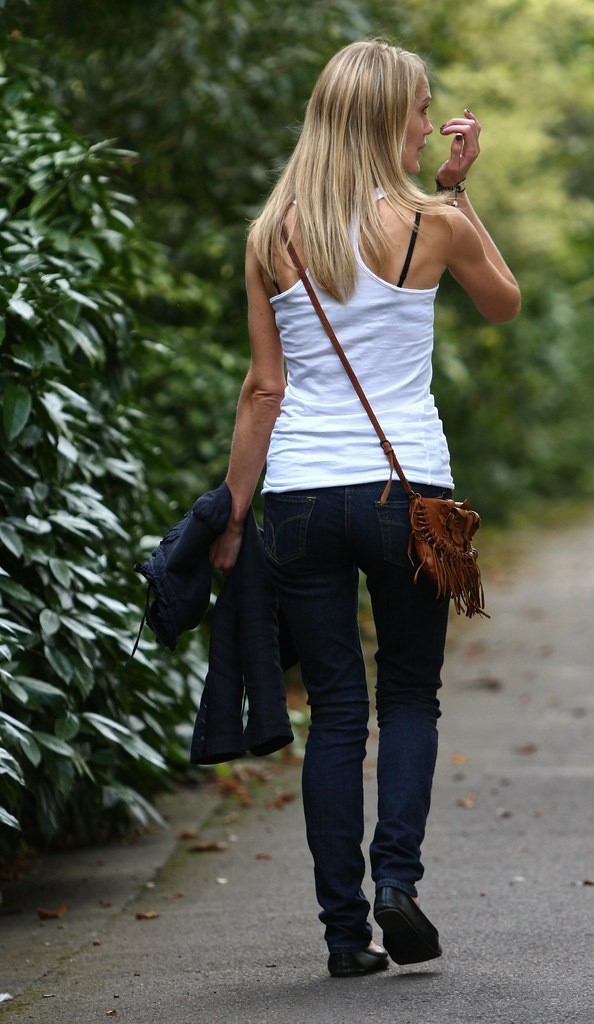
[208,38,519,978]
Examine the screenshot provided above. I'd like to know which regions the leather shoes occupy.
[328,948,389,977]
[373,887,442,966]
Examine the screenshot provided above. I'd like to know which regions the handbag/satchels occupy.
[407,493,492,618]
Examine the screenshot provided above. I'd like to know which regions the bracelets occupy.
[434,177,467,207]
